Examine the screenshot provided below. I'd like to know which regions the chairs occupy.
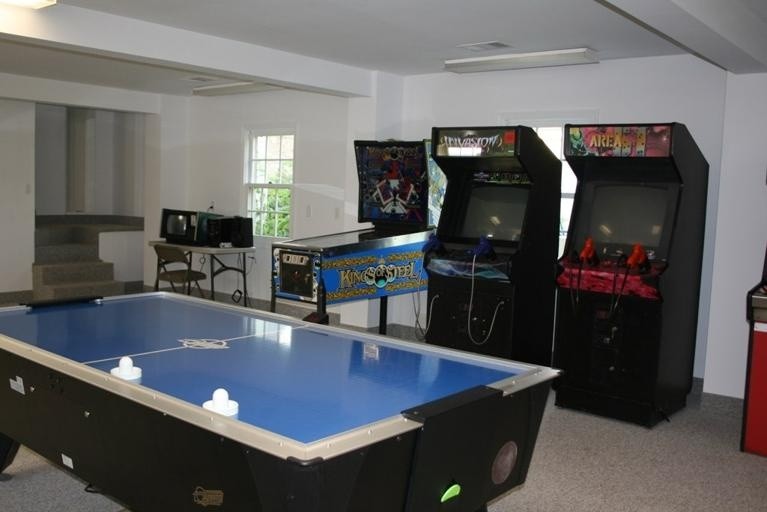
[154,244,206,297]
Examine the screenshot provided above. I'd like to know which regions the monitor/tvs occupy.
[583,183,670,252]
[456,183,535,246]
[205,216,253,249]
[160,208,201,243]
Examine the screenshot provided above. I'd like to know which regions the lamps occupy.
[445,47,600,74]
[193,82,284,97]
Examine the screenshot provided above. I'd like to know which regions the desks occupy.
[149,241,256,307]
[0,291,560,512]
